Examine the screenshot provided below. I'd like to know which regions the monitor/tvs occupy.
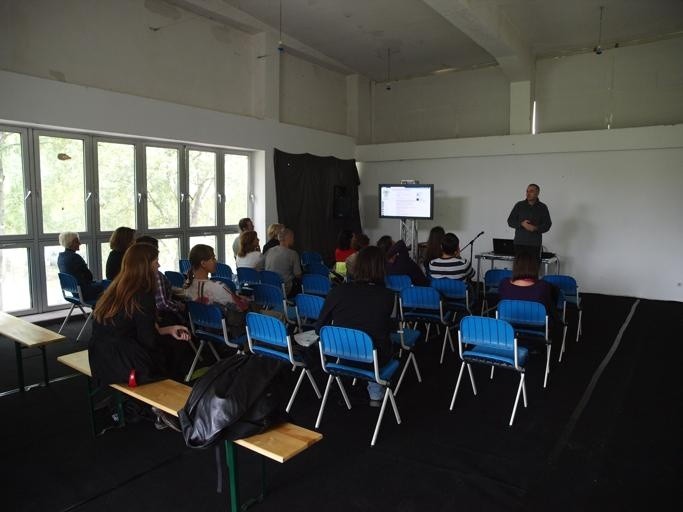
[379,183,435,221]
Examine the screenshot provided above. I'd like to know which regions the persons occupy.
[508,183,552,263]
[499,253,560,328]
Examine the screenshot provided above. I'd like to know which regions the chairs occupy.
[58,252,584,448]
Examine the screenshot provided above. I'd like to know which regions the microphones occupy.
[477,231,485,237]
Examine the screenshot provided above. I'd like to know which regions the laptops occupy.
[493,238,515,255]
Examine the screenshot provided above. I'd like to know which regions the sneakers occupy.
[368,399,382,407]
[152,415,179,431]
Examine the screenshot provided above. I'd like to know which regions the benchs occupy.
[0,307,65,398]
[58,349,324,511]
